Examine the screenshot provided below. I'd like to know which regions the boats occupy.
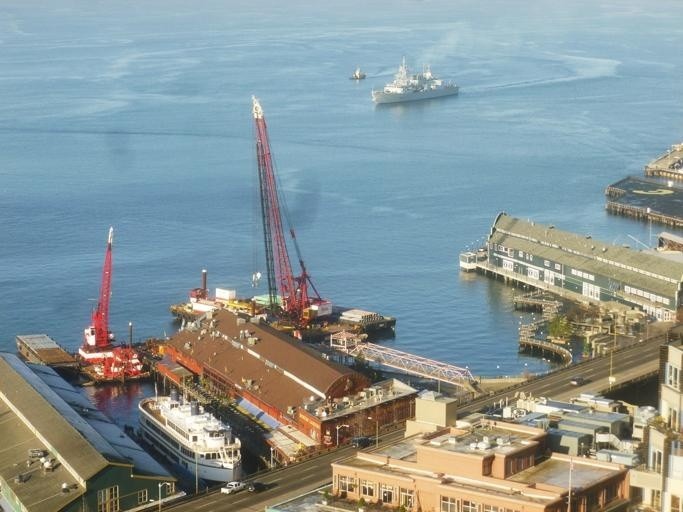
[348,68,366,80]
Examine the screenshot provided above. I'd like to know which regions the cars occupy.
[569,376,584,387]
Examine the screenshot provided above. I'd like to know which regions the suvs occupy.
[350,437,371,449]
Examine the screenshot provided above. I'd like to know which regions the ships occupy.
[72,226,151,387]
[368,55,460,106]
[169,92,397,344]
[136,371,243,486]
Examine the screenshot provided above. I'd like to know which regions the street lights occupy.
[269,443,282,469]
[335,423,350,449]
[157,480,173,512]
[368,416,379,449]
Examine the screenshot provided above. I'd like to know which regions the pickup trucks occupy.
[219,481,268,495]
